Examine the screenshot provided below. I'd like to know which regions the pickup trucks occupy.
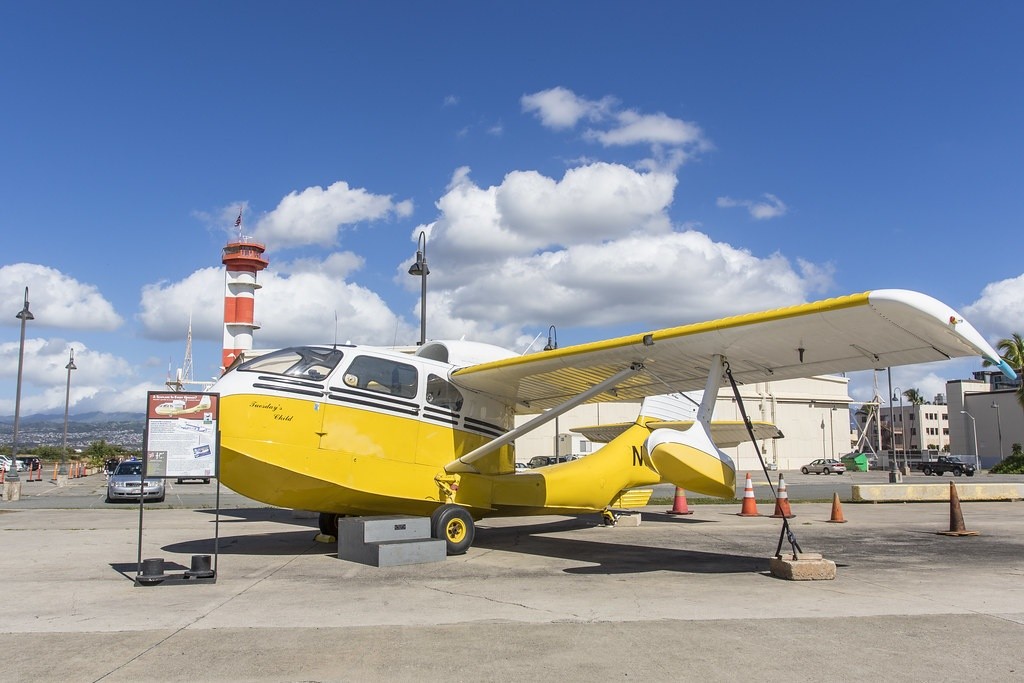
[917,456,976,477]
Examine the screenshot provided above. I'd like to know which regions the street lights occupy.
[892,387,908,467]
[991,400,1003,460]
[544,324,560,466]
[58,348,76,475]
[5,286,35,481]
[961,411,979,471]
[408,231,430,346]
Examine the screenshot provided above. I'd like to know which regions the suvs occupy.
[0,455,23,468]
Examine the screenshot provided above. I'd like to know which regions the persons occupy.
[105,458,122,479]
[130,454,136,461]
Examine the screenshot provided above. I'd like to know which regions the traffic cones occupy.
[937,480,981,537]
[737,474,766,517]
[828,493,847,523]
[768,473,797,519]
[666,484,695,515]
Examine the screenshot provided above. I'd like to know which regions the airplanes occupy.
[202,289,1019,555]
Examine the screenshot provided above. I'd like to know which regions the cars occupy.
[514,462,530,474]
[106,460,165,503]
[800,458,846,475]
[0,460,12,472]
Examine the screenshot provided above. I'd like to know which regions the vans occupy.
[529,457,565,469]
[17,457,42,471]
